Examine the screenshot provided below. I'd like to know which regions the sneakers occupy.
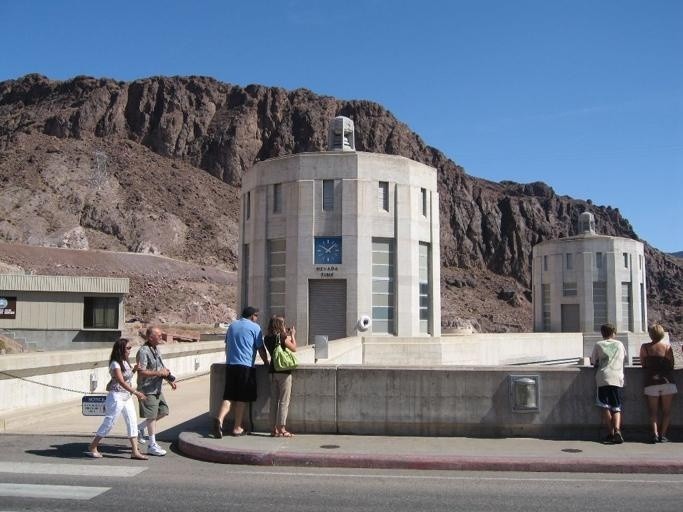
[602,434,615,444]
[614,430,624,444]
[661,435,672,442]
[137,424,146,445]
[147,443,167,456]
[646,435,659,444]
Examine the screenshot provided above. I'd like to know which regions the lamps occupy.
[505,373,541,414]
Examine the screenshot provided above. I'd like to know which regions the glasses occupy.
[125,346,131,350]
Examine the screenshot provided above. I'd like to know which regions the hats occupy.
[600,323,615,338]
[241,307,258,318]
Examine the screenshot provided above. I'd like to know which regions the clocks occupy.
[312,235,342,264]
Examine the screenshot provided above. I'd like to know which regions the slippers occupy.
[213,417,223,438]
[82,445,103,458]
[131,454,149,460]
[231,427,252,436]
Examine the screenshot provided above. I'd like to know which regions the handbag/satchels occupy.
[272,332,298,372]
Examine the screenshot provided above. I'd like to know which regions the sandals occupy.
[279,431,295,437]
[271,424,279,437]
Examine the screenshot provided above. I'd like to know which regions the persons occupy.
[590,324,628,443]
[263,314,297,436]
[212,306,269,440]
[81,339,149,461]
[136,326,177,457]
[639,323,677,444]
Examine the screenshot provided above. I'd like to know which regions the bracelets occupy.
[133,389,137,394]
[158,371,162,378]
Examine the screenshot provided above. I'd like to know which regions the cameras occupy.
[287,328,290,331]
[164,372,175,382]
